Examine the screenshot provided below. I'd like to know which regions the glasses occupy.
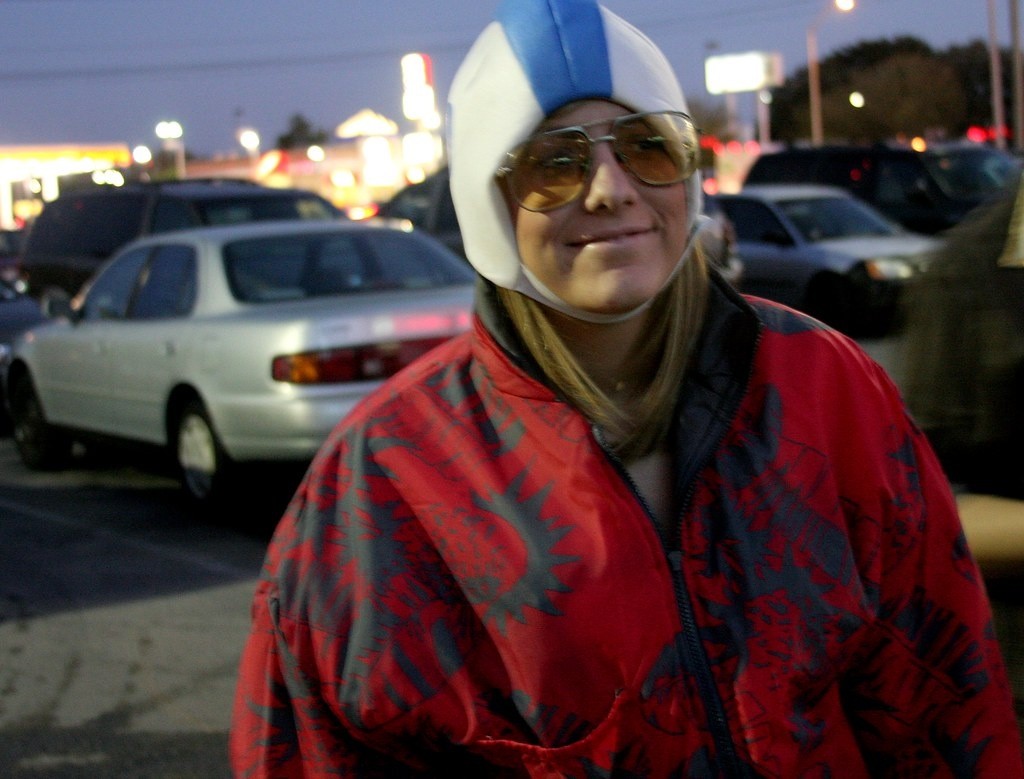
[494,110,704,212]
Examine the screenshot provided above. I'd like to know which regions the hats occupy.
[446,0,711,323]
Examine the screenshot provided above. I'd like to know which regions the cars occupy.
[698,185,947,342]
[1,159,495,330]
[1,222,483,514]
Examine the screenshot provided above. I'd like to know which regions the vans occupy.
[736,145,1023,272]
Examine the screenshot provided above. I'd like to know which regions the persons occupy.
[897,177,1024,737]
[228,0,1024,779]
